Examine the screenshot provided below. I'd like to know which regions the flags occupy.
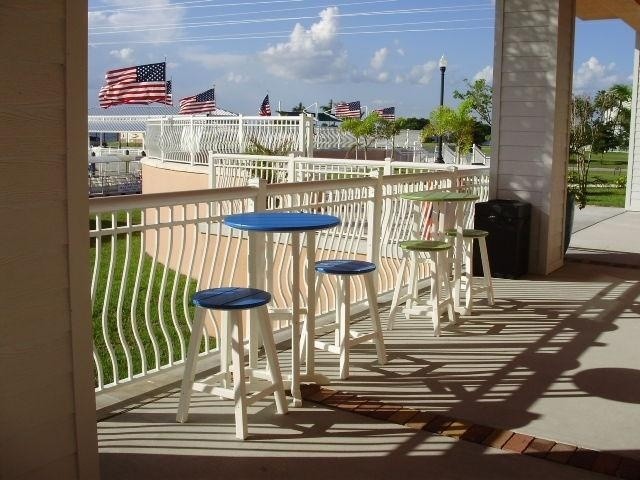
[335,101,360,118]
[374,106,394,122]
[99,61,165,109]
[154,79,171,105]
[258,93,271,116]
[180,88,216,115]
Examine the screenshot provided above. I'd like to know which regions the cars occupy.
[90,136,108,148]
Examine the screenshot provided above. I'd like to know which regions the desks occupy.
[221,209,341,409]
[400,190,480,318]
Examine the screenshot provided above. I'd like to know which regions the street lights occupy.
[435,56,448,164]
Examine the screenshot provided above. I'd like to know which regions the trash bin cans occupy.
[473,198,532,278]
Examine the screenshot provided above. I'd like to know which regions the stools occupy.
[298,258,388,380]
[389,228,496,340]
[176,286,289,442]
